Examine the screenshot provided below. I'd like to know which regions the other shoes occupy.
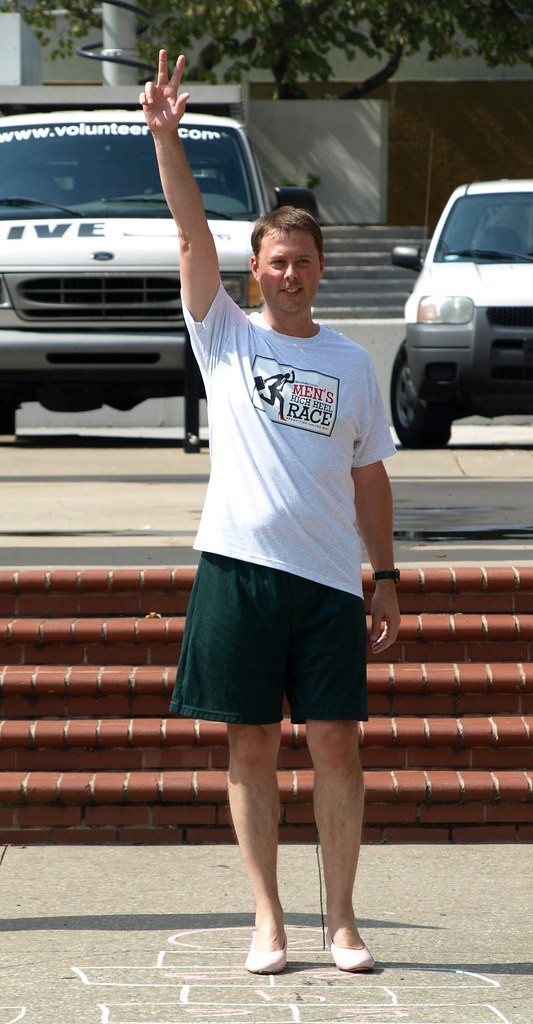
[325,932,375,972]
[246,933,287,974]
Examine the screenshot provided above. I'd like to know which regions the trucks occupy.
[0,80,322,443]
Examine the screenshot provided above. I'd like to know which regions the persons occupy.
[139,49,402,975]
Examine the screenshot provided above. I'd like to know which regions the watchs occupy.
[372,568,400,584]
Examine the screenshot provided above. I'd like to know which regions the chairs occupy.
[0,154,68,202]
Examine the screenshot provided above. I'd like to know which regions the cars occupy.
[389,177,533,452]
